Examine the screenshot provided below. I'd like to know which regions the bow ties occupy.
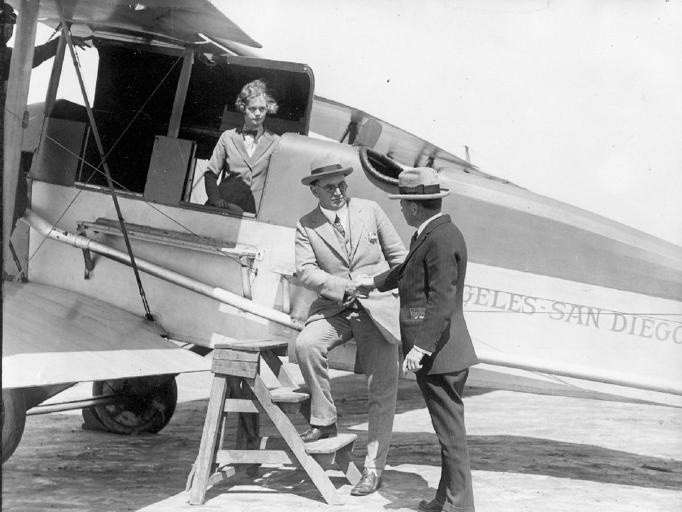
[245,128,258,136]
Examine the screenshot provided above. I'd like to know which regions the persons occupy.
[1,1,94,283]
[294,153,409,497]
[204,79,281,218]
[355,167,481,512]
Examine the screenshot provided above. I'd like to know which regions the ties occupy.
[334,215,344,235]
[412,230,419,240]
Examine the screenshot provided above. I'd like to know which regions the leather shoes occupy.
[418,499,444,512]
[298,421,338,443]
[350,469,383,497]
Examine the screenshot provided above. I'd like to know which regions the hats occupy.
[300,152,353,185]
[388,166,451,201]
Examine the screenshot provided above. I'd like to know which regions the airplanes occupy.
[0,1,682,464]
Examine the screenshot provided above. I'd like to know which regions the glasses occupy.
[315,182,348,192]
